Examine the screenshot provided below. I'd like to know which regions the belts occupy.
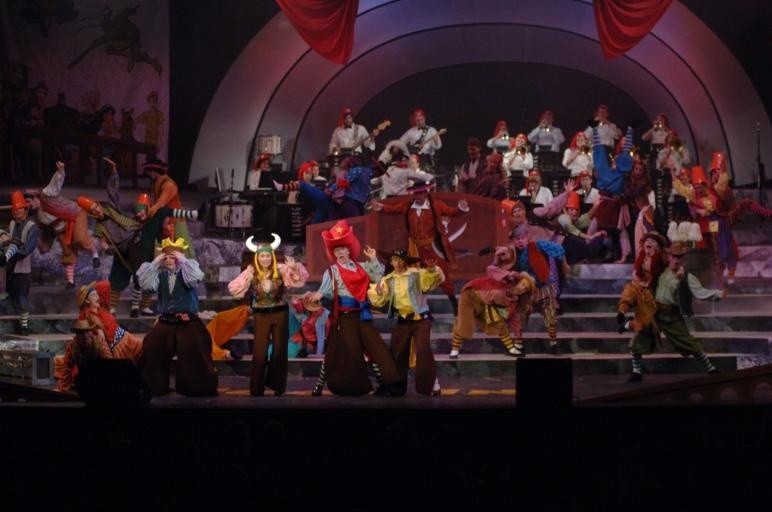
[254,303,289,313]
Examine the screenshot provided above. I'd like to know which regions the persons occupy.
[607,161,655,264]
[0,191,38,335]
[334,154,373,217]
[449,266,536,359]
[367,180,469,316]
[142,158,197,263]
[500,178,578,314]
[300,162,345,222]
[377,139,411,167]
[487,120,514,153]
[689,166,772,286]
[382,155,414,195]
[331,106,377,154]
[226,233,309,396]
[400,109,443,191]
[78,155,209,315]
[311,221,385,399]
[503,134,533,190]
[457,135,487,194]
[26,160,101,290]
[495,224,572,348]
[275,156,328,204]
[587,104,624,191]
[368,248,446,398]
[136,238,219,399]
[519,167,555,206]
[633,231,684,286]
[527,110,566,169]
[77,283,252,362]
[327,150,348,205]
[562,132,594,186]
[708,151,771,220]
[133,191,176,315]
[249,153,280,188]
[577,171,598,213]
[407,152,431,181]
[616,231,729,385]
[60,320,103,396]
[43,87,163,149]
[551,192,608,274]
[643,113,678,177]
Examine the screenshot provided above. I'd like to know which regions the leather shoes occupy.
[198,199,210,220]
[312,385,322,395]
[229,344,243,360]
[628,372,642,383]
[449,356,459,359]
[432,388,441,396]
[618,315,634,334]
[140,310,157,316]
[507,350,527,356]
[130,308,138,318]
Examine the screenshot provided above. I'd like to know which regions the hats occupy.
[500,199,524,216]
[141,152,162,170]
[407,181,434,193]
[156,237,190,255]
[320,220,361,261]
[75,280,97,310]
[467,135,481,146]
[71,319,97,333]
[302,291,321,312]
[709,151,726,175]
[690,165,708,185]
[521,270,538,292]
[643,230,667,248]
[509,221,529,238]
[134,192,150,206]
[134,205,144,214]
[11,191,27,208]
[77,195,95,211]
[497,243,518,270]
[566,191,581,210]
[664,242,689,257]
[392,248,409,257]
